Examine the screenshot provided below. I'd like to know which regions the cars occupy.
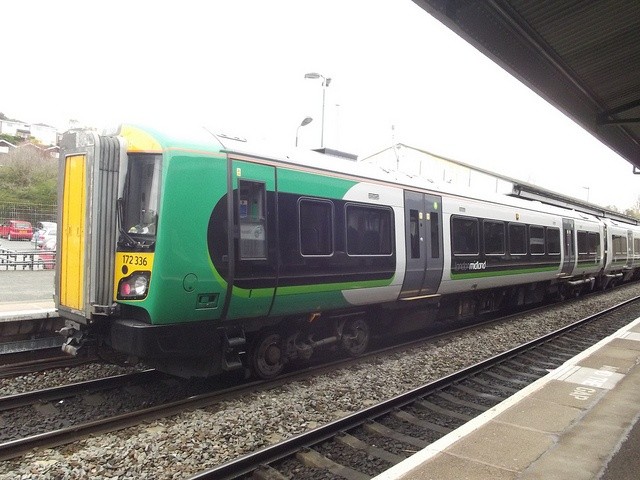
[0,220,33,241]
[38,237,56,270]
[33,229,56,250]
[34,221,57,231]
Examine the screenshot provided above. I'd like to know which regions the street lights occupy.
[304,72,332,147]
[295,117,313,148]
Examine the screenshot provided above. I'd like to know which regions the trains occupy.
[53,115,639,383]
